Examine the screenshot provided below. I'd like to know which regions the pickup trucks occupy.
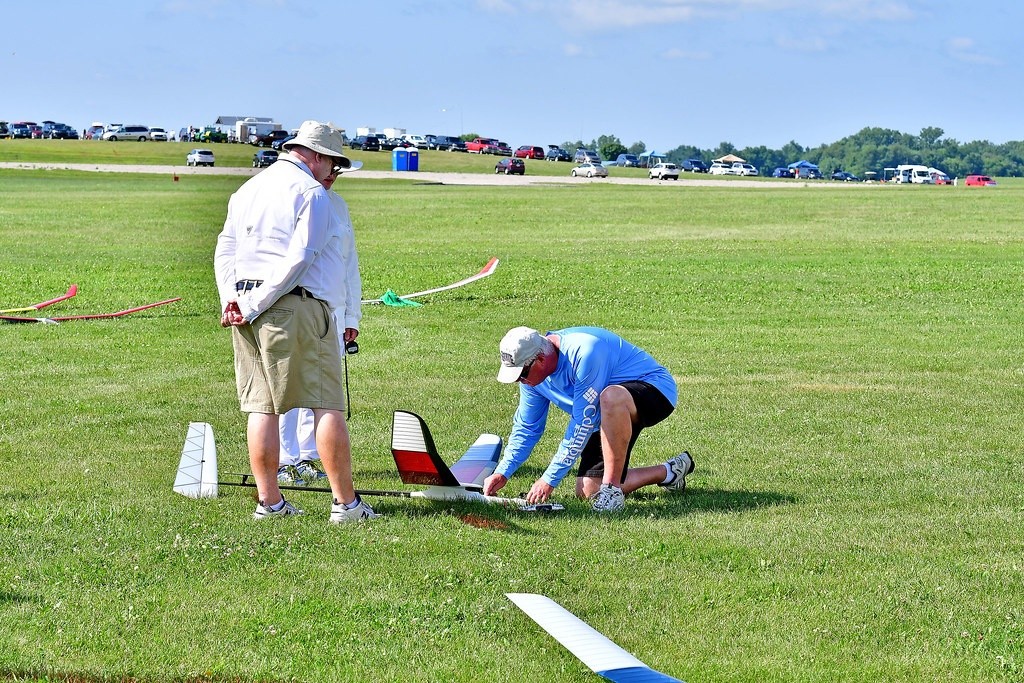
[465,136,513,156]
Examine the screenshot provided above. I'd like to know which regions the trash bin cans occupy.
[393,147,408,171]
[406,147,419,171]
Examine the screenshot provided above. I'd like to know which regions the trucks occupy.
[798,167,824,179]
[179,126,228,145]
[884,164,953,185]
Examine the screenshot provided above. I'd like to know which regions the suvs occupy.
[349,132,467,152]
[965,175,996,187]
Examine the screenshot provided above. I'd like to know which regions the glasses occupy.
[330,164,341,175]
[516,354,545,383]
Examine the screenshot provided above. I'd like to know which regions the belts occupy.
[237,281,312,298]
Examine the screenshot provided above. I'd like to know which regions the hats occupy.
[497,327,541,383]
[282,120,352,169]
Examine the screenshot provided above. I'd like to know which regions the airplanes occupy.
[171,409,529,507]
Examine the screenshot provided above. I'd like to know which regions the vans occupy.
[514,145,603,163]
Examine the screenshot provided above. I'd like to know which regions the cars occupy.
[831,173,862,181]
[252,150,279,168]
[251,129,297,150]
[494,157,525,175]
[0,120,170,142]
[772,167,793,178]
[681,159,759,177]
[570,163,609,178]
[647,163,680,180]
[187,149,216,167]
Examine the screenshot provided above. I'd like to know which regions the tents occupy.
[717,154,746,163]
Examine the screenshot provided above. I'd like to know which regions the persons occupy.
[278,161,363,485]
[187,126,195,141]
[214,121,380,524]
[483,326,695,513]
[169,129,176,142]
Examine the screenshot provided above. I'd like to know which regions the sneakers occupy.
[666,451,695,492]
[277,464,307,487]
[329,493,378,523]
[296,460,327,480]
[593,484,625,512]
[253,493,301,520]
